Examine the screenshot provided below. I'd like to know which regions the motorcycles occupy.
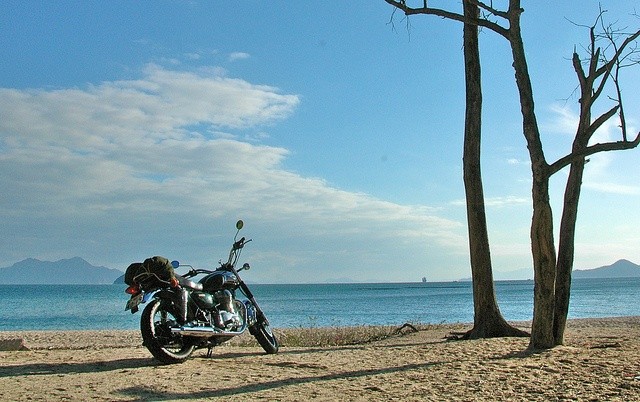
[125,219,278,364]
